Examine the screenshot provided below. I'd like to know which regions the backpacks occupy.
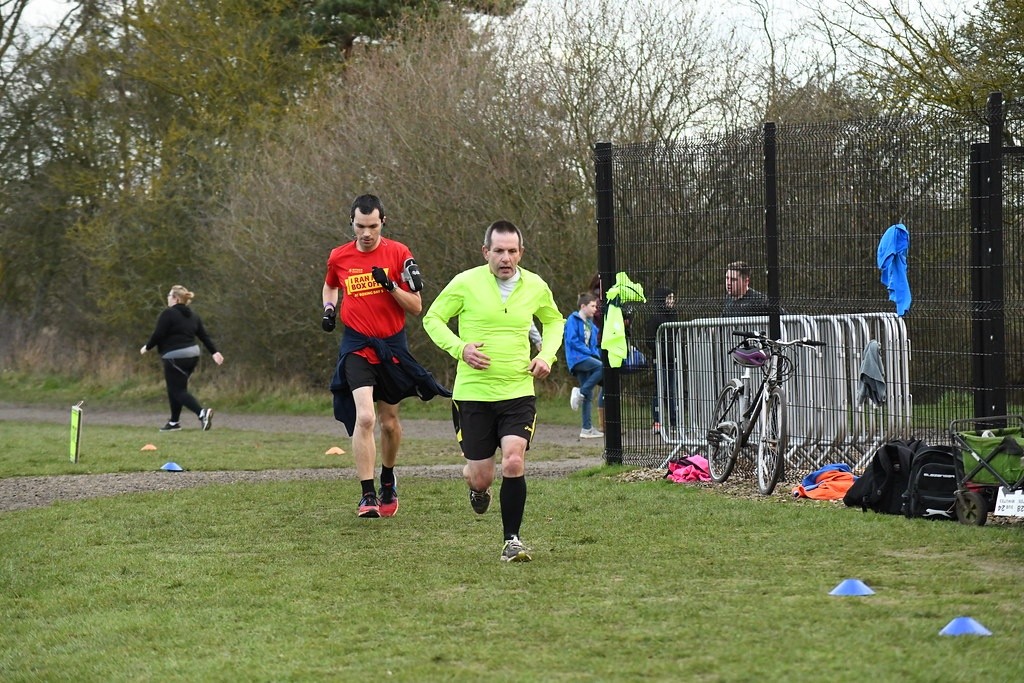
[843,436,963,519]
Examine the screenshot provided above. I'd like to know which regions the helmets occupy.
[731,348,769,368]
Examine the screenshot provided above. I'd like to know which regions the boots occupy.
[598,406,627,435]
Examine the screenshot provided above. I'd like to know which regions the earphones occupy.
[382,223,385,226]
[350,222,353,226]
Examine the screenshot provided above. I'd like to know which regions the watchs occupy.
[387,282,398,292]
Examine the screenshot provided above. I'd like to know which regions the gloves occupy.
[372,265,394,292]
[321,310,337,332]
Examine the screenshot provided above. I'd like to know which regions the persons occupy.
[530,321,542,352]
[322,194,452,516]
[140,285,224,431]
[564,271,646,438]
[423,221,563,562]
[722,261,785,317]
[646,288,678,435]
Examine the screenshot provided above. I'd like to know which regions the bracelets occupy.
[323,302,336,312]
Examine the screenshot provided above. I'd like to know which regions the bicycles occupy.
[706,330,827,496]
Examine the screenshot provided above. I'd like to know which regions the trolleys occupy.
[949,414,1023,526]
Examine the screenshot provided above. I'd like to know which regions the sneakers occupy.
[199,408,214,430]
[469,482,491,514]
[579,427,604,438]
[376,471,399,518]
[159,422,182,432]
[499,537,532,563]
[571,386,584,413]
[358,493,382,517]
